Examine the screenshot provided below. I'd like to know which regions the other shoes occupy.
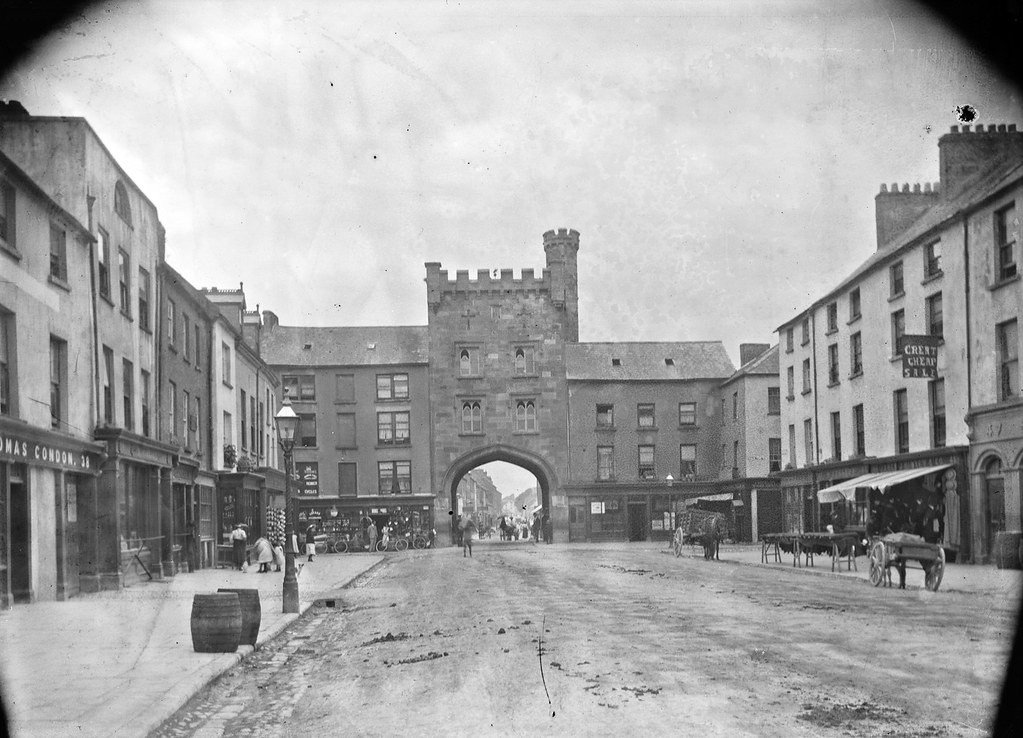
[263,570,267,573]
[239,569,243,571]
[308,559,313,561]
[257,570,262,573]
[273,569,280,572]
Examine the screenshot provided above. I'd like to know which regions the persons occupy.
[367,520,378,552]
[499,516,507,531]
[532,513,540,541]
[455,515,464,541]
[522,522,528,538]
[462,518,475,557]
[306,525,316,562]
[230,523,247,571]
[255,536,273,573]
[381,524,390,551]
[271,542,283,572]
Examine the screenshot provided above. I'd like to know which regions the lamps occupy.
[667,473,673,487]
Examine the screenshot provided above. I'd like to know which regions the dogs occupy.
[294,564,304,576]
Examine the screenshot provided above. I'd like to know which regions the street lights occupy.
[271,388,302,613]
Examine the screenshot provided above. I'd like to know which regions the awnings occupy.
[817,464,953,502]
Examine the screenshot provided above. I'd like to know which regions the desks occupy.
[217,544,251,570]
[760,532,855,575]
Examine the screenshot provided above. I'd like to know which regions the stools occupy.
[191,593,243,653]
[217,589,261,644]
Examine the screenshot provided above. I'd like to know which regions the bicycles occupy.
[315,538,348,555]
[407,532,427,550]
[375,535,408,553]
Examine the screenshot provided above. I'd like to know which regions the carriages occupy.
[499,516,519,541]
[672,508,729,560]
[862,514,946,592]
